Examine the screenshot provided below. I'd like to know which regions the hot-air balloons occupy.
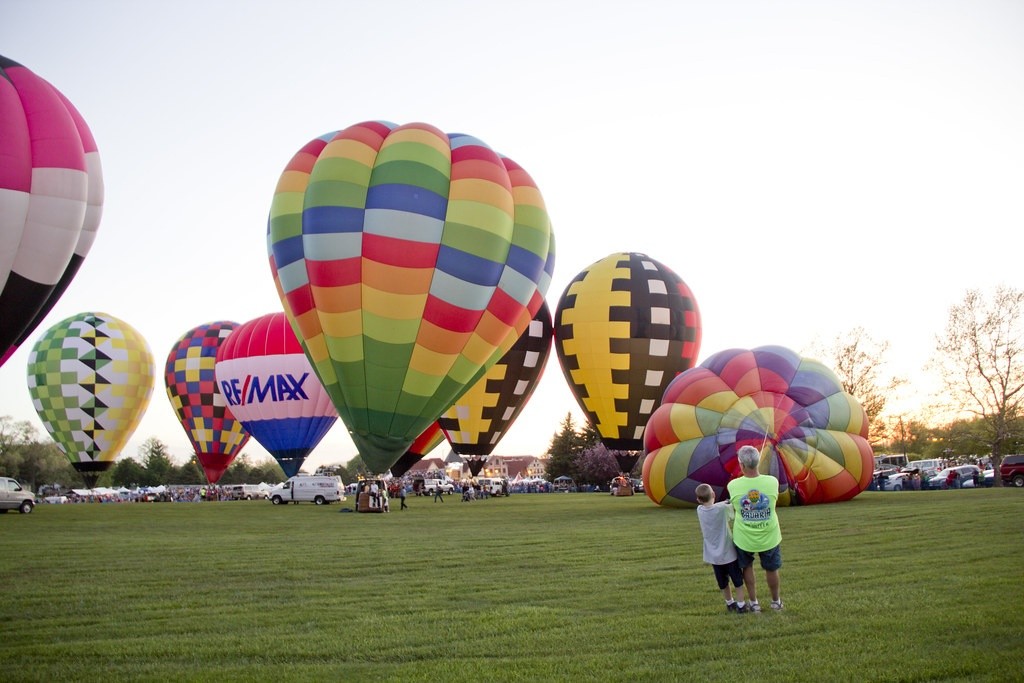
[642,344,875,507]
[213,311,341,482]
[264,119,556,517]
[0,54,104,365]
[388,292,553,491]
[25,311,155,502]
[551,250,704,497]
[163,318,253,502]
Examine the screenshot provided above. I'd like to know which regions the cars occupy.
[871,454,947,491]
[258,488,270,499]
[928,465,980,490]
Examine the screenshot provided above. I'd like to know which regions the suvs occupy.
[1000,454,1024,487]
[0,477,36,513]
[412,478,455,496]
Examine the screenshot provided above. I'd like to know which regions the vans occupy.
[267,475,345,505]
[477,478,511,496]
[354,477,390,513]
[231,485,260,500]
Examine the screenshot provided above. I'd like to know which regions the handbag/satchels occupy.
[440,490,443,493]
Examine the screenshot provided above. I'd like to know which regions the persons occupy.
[695,484,753,612]
[727,446,782,611]
[65,471,643,512]
[871,454,993,491]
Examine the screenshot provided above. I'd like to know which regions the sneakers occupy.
[749,603,761,612]
[770,601,784,611]
[727,602,749,614]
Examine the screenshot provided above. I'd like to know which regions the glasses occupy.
[737,456,741,464]
